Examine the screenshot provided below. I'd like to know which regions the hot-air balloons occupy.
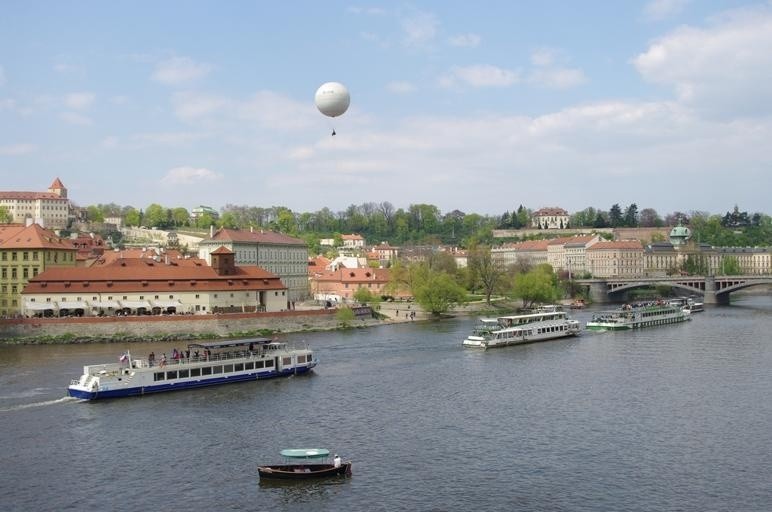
[314,82,351,135]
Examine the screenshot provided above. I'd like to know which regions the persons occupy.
[406,311,416,320]
[249,342,256,358]
[621,296,667,311]
[148,346,211,366]
[379,305,380,310]
[334,455,341,468]
[124,311,128,316]
[117,312,120,316]
[408,304,410,309]
[396,309,398,316]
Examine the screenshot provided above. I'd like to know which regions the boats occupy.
[68,339,320,400]
[586,297,703,330]
[257,448,353,480]
[462,299,586,348]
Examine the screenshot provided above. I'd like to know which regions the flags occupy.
[120,349,129,364]
